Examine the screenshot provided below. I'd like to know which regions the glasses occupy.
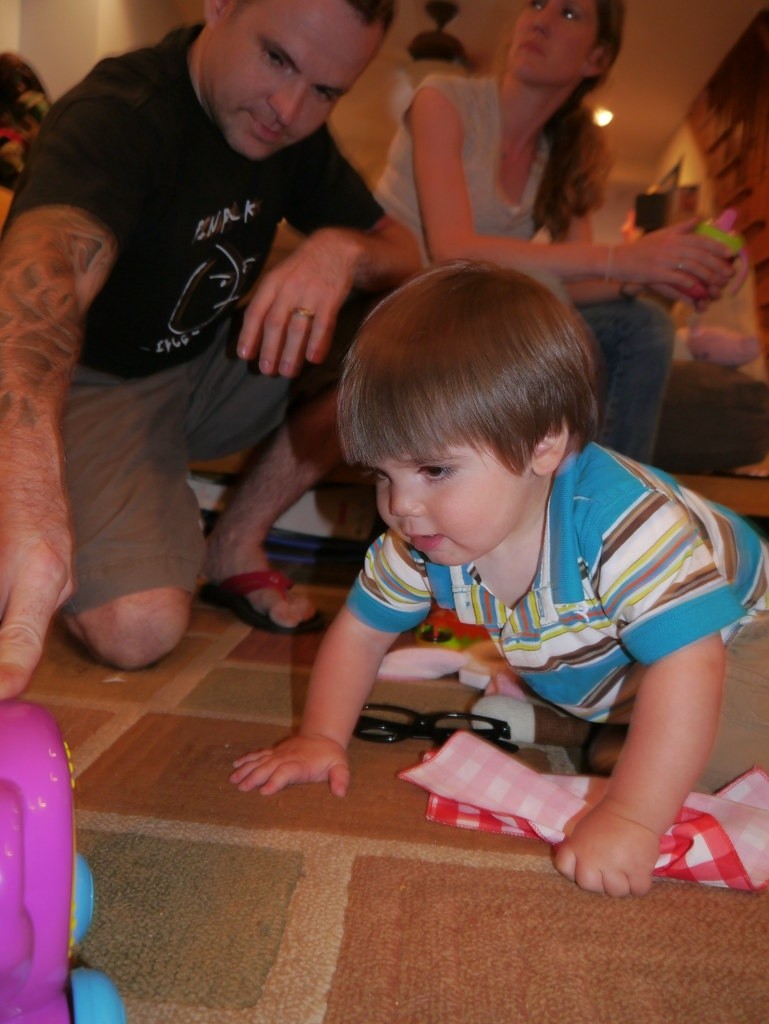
[352,704,520,753]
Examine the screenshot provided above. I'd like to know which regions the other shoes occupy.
[578,722,629,776]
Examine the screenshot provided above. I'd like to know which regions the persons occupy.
[0,50,52,237]
[0,0,425,701]
[227,259,769,900]
[374,0,740,464]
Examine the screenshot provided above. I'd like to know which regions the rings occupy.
[296,308,313,317]
[676,262,683,270]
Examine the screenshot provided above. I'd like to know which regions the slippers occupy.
[198,569,326,634]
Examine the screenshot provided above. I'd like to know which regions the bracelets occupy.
[594,244,615,276]
